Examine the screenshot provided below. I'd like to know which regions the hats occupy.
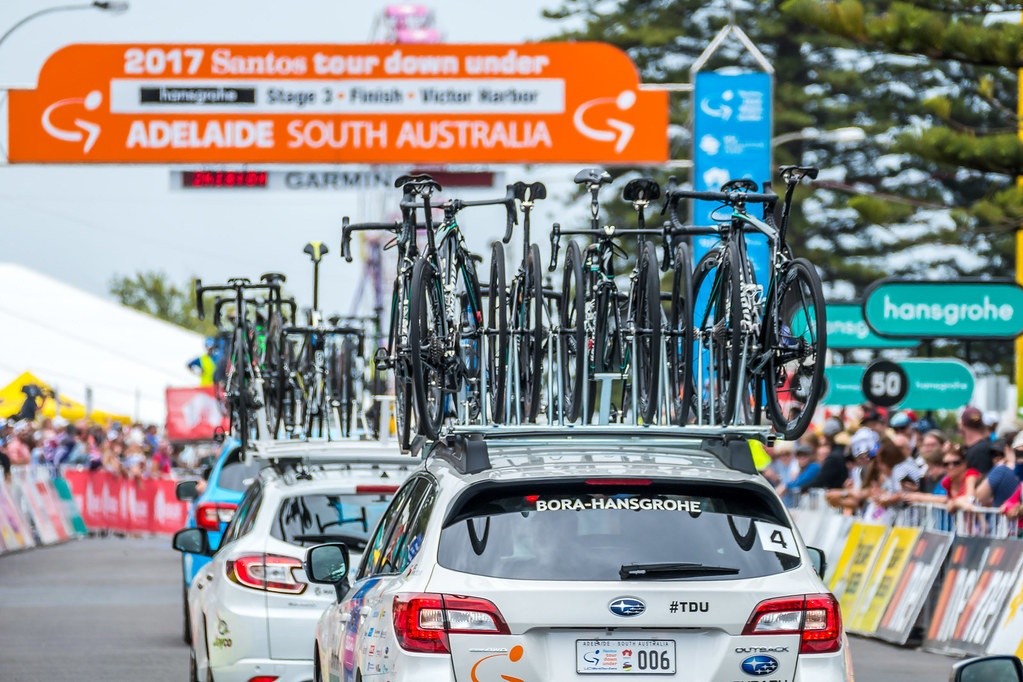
[889,412,910,428]
[963,407,982,428]
[851,427,879,461]
[795,446,811,454]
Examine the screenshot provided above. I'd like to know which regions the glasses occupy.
[942,460,961,467]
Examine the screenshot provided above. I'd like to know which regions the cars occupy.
[169,452,427,682]
[302,430,858,682]
[172,434,396,646]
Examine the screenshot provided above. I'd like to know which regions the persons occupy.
[773,404,1023,540]
[0,413,232,487]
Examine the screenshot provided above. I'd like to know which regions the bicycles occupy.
[190,162,829,460]
